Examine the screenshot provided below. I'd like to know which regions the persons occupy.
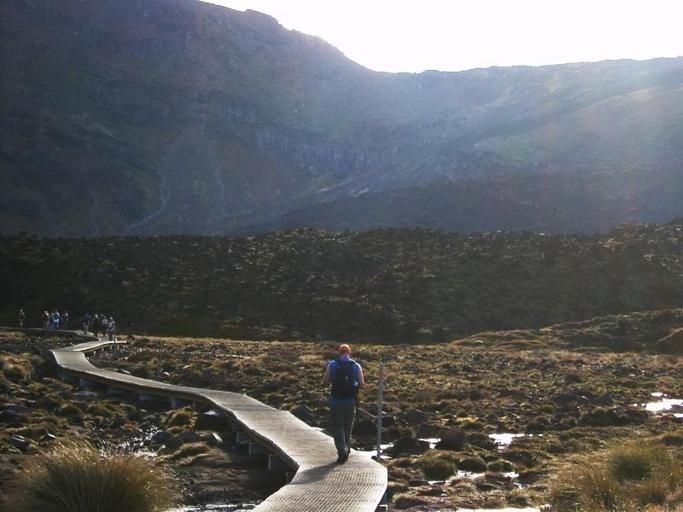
[41,308,115,341]
[322,343,364,463]
[16,308,24,327]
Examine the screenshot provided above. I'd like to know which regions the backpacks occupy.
[330,358,358,398]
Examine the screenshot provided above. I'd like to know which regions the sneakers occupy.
[336,453,349,465]
